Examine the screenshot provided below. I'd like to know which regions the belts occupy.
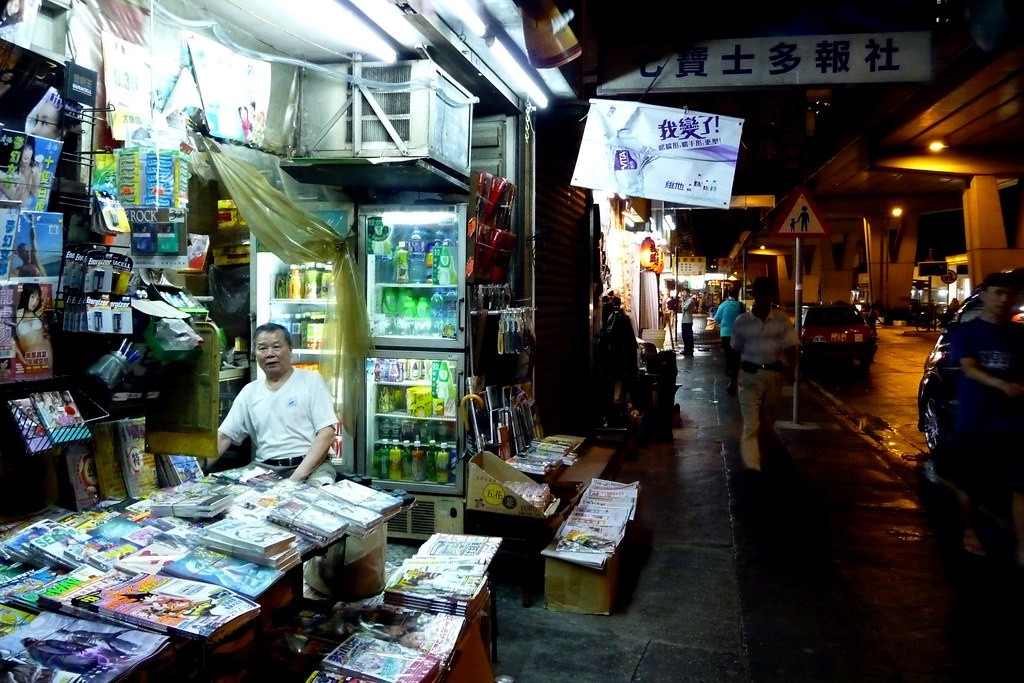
[740,362,780,370]
[263,456,303,466]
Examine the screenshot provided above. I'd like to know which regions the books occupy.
[7,398,53,455]
[504,436,579,476]
[0,454,504,682]
[0,127,66,386]
[368,614,443,652]
[31,391,91,445]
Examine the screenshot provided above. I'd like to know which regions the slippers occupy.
[963,542,988,559]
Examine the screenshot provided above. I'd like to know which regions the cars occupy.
[797,300,878,371]
[916,272,1024,498]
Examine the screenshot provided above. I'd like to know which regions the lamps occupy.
[518,0,582,69]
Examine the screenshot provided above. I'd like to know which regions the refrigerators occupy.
[256,202,469,496]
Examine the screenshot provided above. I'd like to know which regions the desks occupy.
[119,485,498,683]
[467,428,628,607]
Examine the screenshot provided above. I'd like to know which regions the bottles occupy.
[372,230,457,336]
[270,260,334,348]
[379,438,449,484]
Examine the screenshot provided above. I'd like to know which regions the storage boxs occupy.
[542,542,621,616]
[466,451,557,519]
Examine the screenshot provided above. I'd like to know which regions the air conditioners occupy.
[300,59,475,180]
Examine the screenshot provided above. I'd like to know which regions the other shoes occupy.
[680,351,694,355]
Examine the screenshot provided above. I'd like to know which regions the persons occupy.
[4,284,53,373]
[679,288,694,355]
[714,289,745,377]
[862,304,882,333]
[32,392,83,428]
[0,71,14,95]
[602,291,624,333]
[11,400,39,429]
[917,272,1024,564]
[0,137,42,201]
[726,276,799,474]
[942,298,960,327]
[29,97,64,141]
[9,214,47,278]
[667,290,707,326]
[195,323,339,487]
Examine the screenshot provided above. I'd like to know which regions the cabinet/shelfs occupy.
[0,103,115,457]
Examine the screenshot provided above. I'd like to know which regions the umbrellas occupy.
[460,393,484,463]
